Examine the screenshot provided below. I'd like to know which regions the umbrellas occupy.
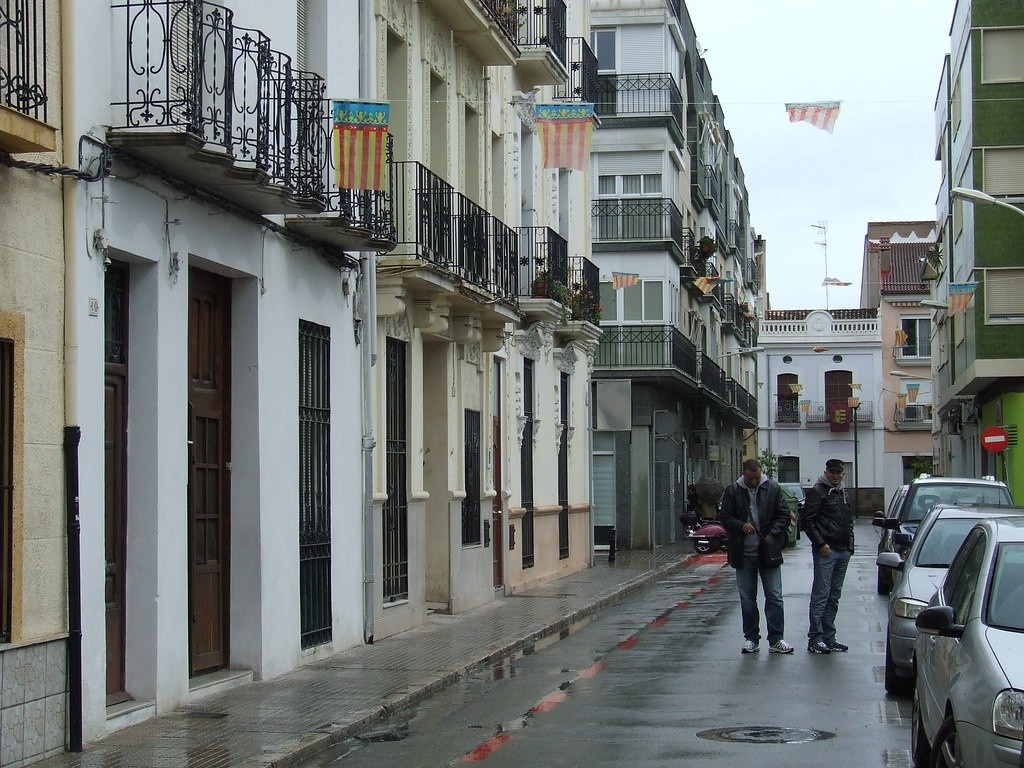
[696,477,723,503]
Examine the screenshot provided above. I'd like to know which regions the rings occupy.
[749,530,751,532]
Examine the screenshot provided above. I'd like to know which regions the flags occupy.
[611,272,639,289]
[897,393,907,408]
[822,277,853,286]
[533,103,596,170]
[785,103,840,135]
[906,384,920,403]
[847,397,860,407]
[801,399,811,412]
[693,276,734,295]
[894,330,909,348]
[331,101,389,191]
[947,283,979,317]
[850,384,863,391]
[789,383,803,394]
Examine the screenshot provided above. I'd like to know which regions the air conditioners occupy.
[905,406,925,421]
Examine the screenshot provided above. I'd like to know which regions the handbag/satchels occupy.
[759,538,783,567]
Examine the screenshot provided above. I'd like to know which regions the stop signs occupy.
[983,427,1008,452]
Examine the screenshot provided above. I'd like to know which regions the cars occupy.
[777,481,807,511]
[911,514,1023,768]
[873,505,1024,699]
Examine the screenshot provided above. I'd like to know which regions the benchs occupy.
[594,525,616,562]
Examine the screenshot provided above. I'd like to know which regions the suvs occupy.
[871,473,1015,596]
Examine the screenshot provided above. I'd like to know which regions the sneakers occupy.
[807,641,832,654]
[768,640,794,653]
[742,640,760,652]
[825,641,848,651]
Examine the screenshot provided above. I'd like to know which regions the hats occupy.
[826,459,844,472]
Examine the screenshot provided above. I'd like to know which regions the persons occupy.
[802,460,855,655]
[716,459,794,654]
[686,484,718,519]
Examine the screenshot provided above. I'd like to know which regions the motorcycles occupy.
[679,513,728,553]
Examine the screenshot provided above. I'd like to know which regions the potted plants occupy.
[531,256,603,329]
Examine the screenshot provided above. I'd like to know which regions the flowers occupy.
[738,302,750,314]
[742,314,756,323]
